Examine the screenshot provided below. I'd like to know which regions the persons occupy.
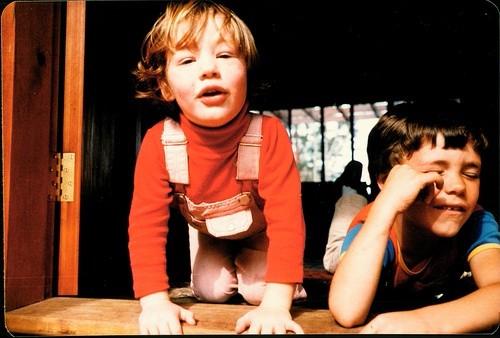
[323,102,500,334]
[127,0,307,335]
